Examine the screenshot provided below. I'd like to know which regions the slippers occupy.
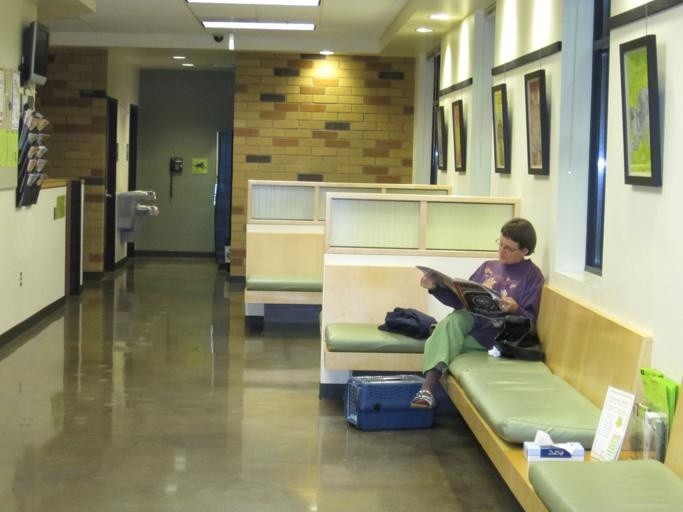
[410,387,439,411]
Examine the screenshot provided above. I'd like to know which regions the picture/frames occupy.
[436,106,447,170]
[492,83,511,173]
[523,68,550,176]
[619,32,662,186]
[452,100,466,172]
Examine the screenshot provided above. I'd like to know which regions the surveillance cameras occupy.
[213,35,225,42]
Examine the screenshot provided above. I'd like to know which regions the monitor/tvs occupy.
[21,21,50,86]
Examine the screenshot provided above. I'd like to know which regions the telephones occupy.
[170,157,184,173]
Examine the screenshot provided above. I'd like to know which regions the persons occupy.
[410,217,545,409]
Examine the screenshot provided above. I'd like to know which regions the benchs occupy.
[244,177,683,512]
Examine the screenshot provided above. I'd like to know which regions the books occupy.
[415,263,507,321]
[22,108,51,188]
[590,366,679,462]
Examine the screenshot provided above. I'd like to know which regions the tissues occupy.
[522,428,585,463]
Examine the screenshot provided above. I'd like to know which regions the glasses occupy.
[495,238,519,255]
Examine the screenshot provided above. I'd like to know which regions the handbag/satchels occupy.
[495,313,544,360]
